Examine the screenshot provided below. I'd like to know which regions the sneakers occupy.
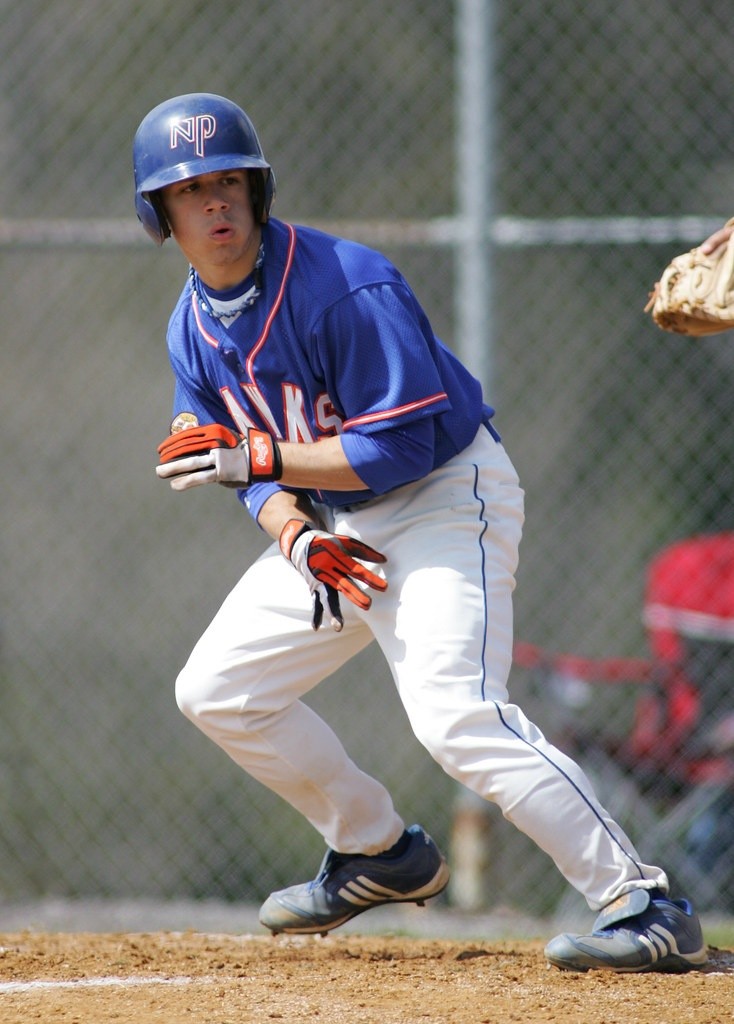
[259,824,449,938]
[544,888,709,972]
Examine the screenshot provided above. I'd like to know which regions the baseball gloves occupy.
[642,217,733,334]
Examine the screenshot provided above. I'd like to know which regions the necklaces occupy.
[188,243,265,319]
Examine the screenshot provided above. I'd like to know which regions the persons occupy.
[637,492,732,914]
[132,93,707,973]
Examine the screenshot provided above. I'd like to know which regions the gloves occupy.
[156,424,282,491]
[279,519,388,632]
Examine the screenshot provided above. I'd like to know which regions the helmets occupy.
[133,93,276,247]
